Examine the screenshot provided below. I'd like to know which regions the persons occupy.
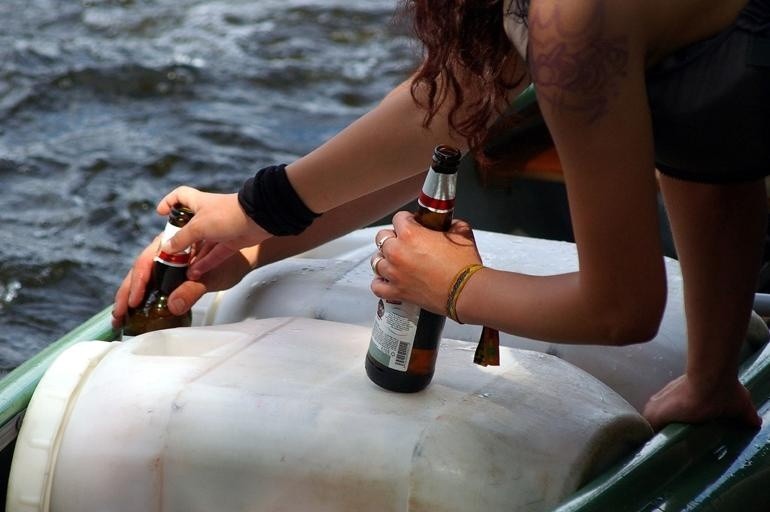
[112,1,770,427]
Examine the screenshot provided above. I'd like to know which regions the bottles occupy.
[364,144,463,394]
[119,204,193,336]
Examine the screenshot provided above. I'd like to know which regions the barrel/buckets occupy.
[6,314,654,512]
[191,225,770,427]
